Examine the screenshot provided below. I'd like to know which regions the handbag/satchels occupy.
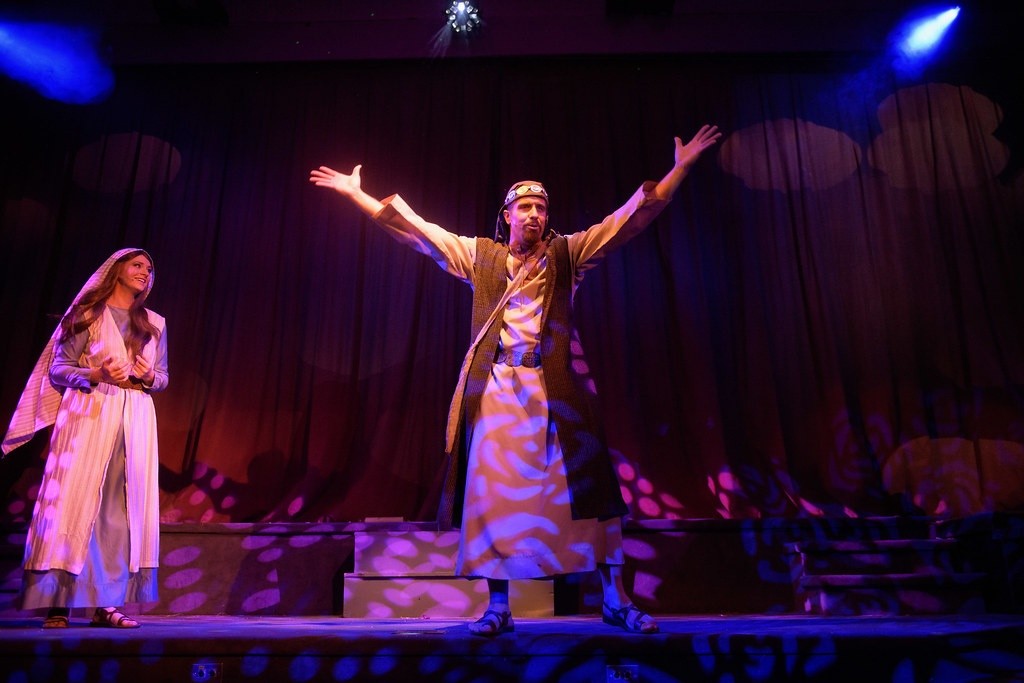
[445,349,475,454]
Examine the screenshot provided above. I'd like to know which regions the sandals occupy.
[467,609,514,634]
[602,601,660,634]
[44,611,69,629]
[89,606,140,628]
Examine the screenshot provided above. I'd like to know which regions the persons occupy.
[0,249,167,629]
[310,124,723,634]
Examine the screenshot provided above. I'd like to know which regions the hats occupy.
[505,181,549,206]
